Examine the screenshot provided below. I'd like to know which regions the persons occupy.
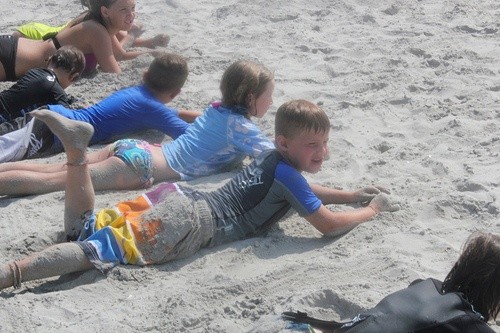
[0,44,86,126]
[0,0,170,83]
[0,60,276,197]
[0,99,401,290]
[0,53,203,163]
[345,233,500,333]
